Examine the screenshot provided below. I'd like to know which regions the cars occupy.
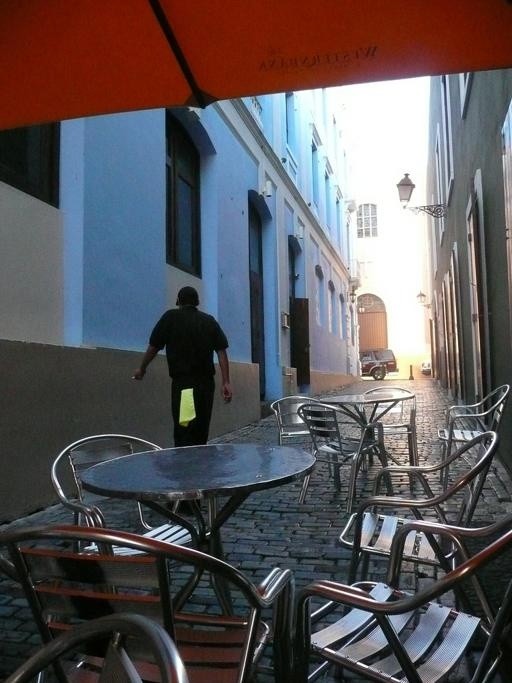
[420,359,433,377]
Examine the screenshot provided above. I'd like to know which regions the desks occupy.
[319,393,416,479]
[80,442,317,617]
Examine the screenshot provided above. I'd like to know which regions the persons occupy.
[133,286,233,517]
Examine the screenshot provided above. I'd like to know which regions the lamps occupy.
[295,226,303,239]
[349,289,356,303]
[262,180,272,198]
[417,290,432,309]
[359,302,365,313]
[395,173,446,218]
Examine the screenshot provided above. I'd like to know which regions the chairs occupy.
[338,430,498,617]
[361,386,419,469]
[1,522,297,682]
[436,385,510,492]
[296,403,393,514]
[51,434,237,606]
[270,396,332,478]
[295,511,511,681]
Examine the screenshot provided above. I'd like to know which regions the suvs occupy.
[358,348,401,380]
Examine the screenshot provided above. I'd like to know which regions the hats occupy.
[176,287,199,306]
